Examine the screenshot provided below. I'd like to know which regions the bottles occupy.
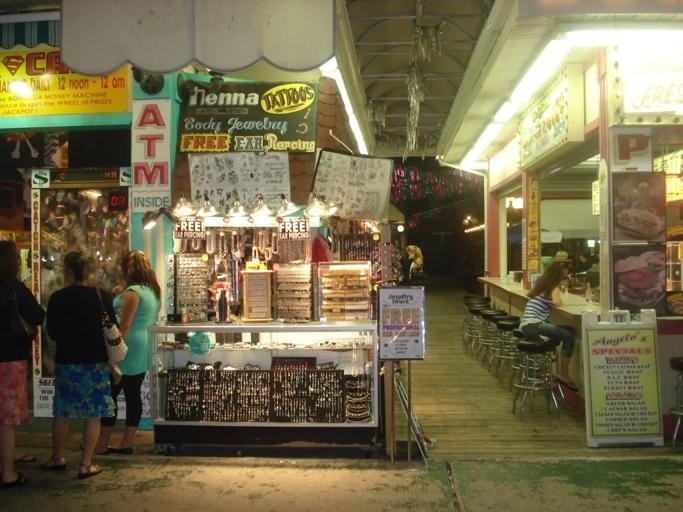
[585,282,592,305]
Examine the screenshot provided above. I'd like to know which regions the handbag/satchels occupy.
[102,323,128,362]
[17,312,37,339]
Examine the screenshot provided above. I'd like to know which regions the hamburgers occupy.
[615,208,662,239]
[615,251,667,310]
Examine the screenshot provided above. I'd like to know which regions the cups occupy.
[560,285,568,295]
[513,272,522,282]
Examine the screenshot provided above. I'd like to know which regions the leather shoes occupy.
[111,446,132,453]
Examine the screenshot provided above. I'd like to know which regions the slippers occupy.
[1,474,27,488]
[557,376,578,390]
[13,453,33,461]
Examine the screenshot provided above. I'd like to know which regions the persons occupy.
[0,239,45,487]
[42,251,121,478]
[81,249,161,455]
[406,245,424,279]
[521,262,578,393]
[575,252,592,271]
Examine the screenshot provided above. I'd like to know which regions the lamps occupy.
[142,187,343,230]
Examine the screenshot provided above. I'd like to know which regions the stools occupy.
[461,295,565,421]
[670,357,683,447]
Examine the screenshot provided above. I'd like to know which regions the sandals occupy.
[41,457,64,469]
[79,464,102,478]
